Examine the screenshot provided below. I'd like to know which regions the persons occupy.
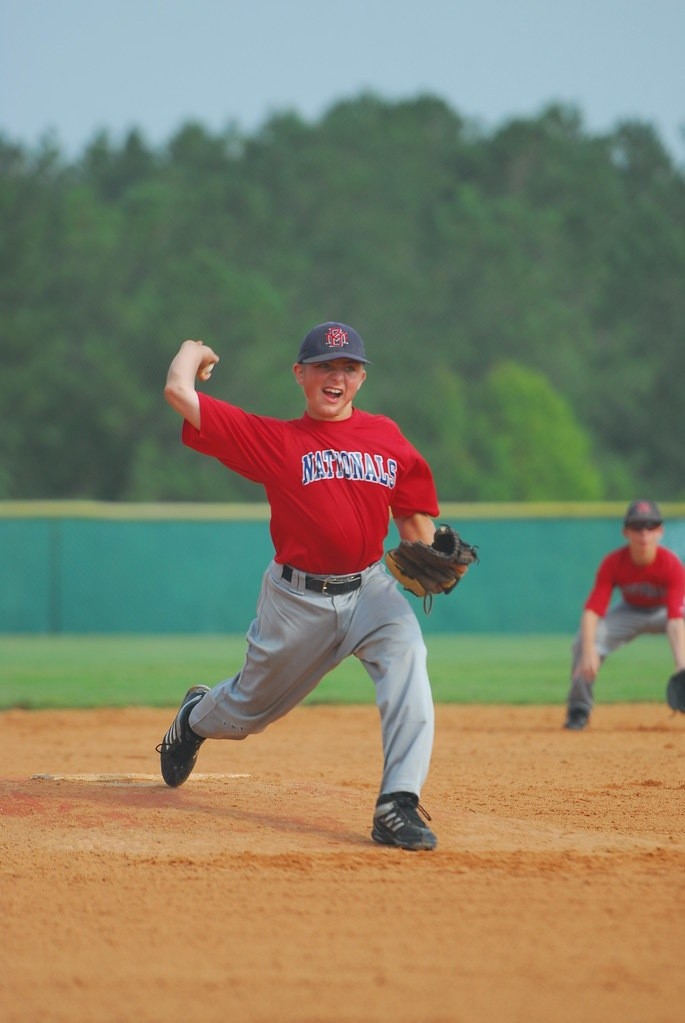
[160,322,446,851]
[558,500,684,729]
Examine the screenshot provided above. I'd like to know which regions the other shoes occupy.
[562,708,591,732]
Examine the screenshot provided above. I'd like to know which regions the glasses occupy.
[625,520,663,530]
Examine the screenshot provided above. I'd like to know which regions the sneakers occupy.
[370,795,439,850]
[159,685,211,788]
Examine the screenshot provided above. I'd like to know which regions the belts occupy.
[281,565,363,595]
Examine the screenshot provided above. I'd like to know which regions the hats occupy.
[624,499,664,530]
[296,321,373,365]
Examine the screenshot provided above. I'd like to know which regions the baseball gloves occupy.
[384,524,480,616]
[665,669,685,720]
